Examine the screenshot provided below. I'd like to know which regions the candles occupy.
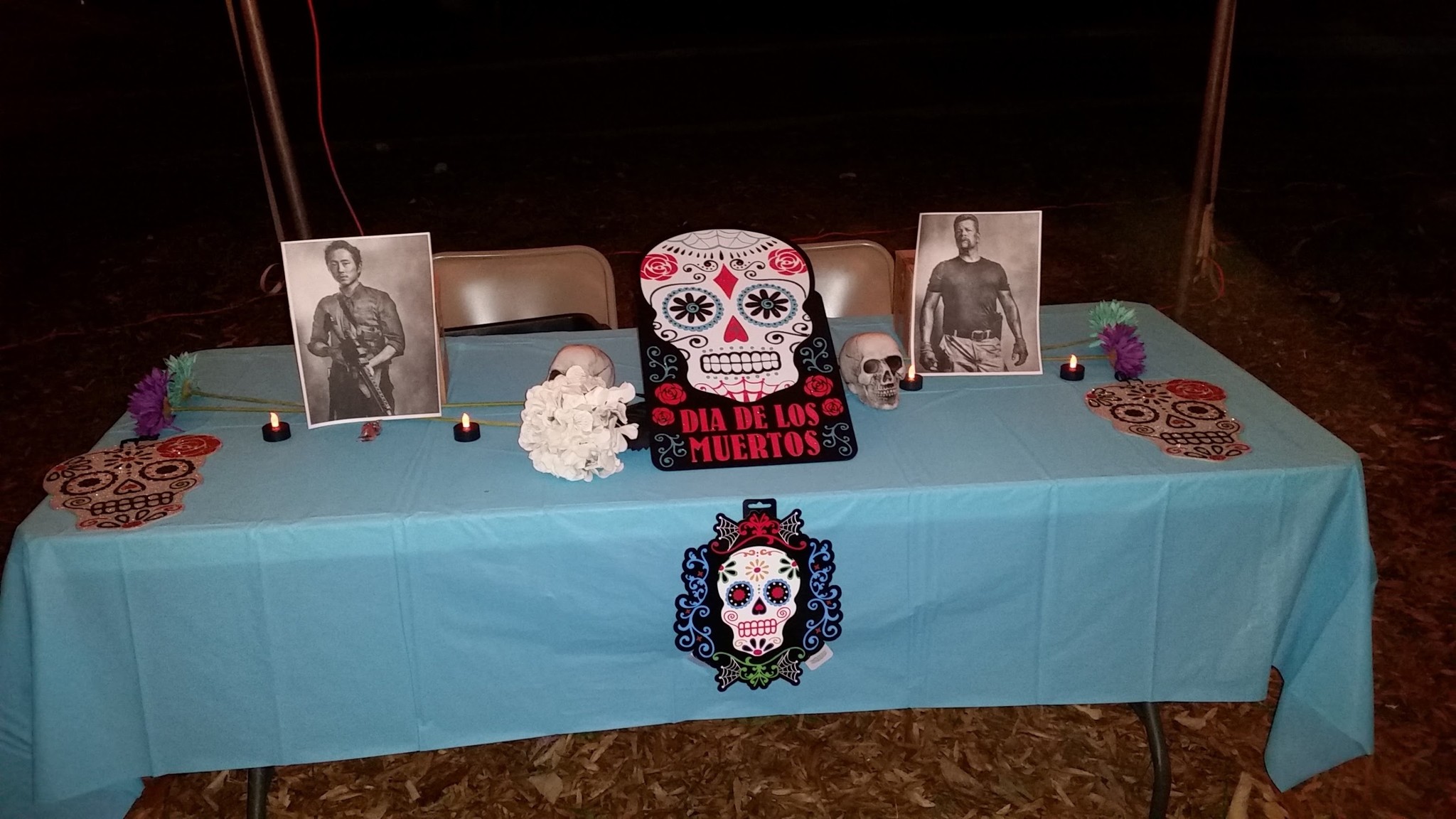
[263,406,292,439]
[1058,352,1087,378]
[900,363,922,391]
[449,412,481,444]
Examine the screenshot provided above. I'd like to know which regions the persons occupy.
[920,214,1029,372]
[308,240,407,421]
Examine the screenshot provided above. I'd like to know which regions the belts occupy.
[943,327,999,342]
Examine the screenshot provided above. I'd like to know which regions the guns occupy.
[326,312,394,416]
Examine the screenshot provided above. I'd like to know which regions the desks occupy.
[0,301,1378,818]
